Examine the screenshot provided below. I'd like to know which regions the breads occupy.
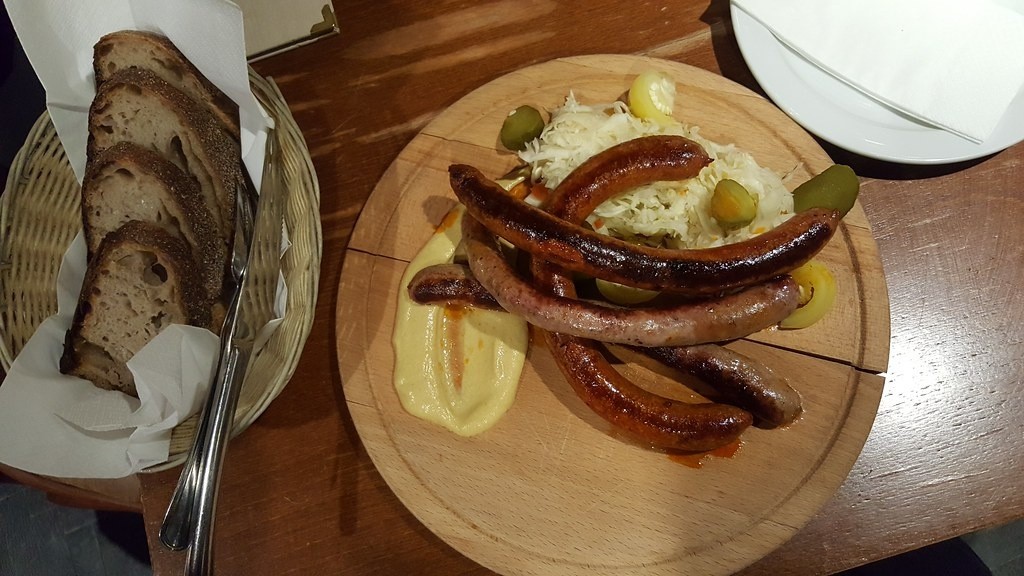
[55,29,243,399]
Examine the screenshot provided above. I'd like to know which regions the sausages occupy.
[407,134,844,458]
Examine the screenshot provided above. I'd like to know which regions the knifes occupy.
[182,125,283,575]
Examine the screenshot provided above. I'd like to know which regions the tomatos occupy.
[627,69,677,128]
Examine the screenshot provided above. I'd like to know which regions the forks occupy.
[158,176,262,551]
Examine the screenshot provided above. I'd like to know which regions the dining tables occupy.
[140,0,1024,576]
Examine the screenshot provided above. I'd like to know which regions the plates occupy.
[334,51,891,572]
[730,0,1024,165]
